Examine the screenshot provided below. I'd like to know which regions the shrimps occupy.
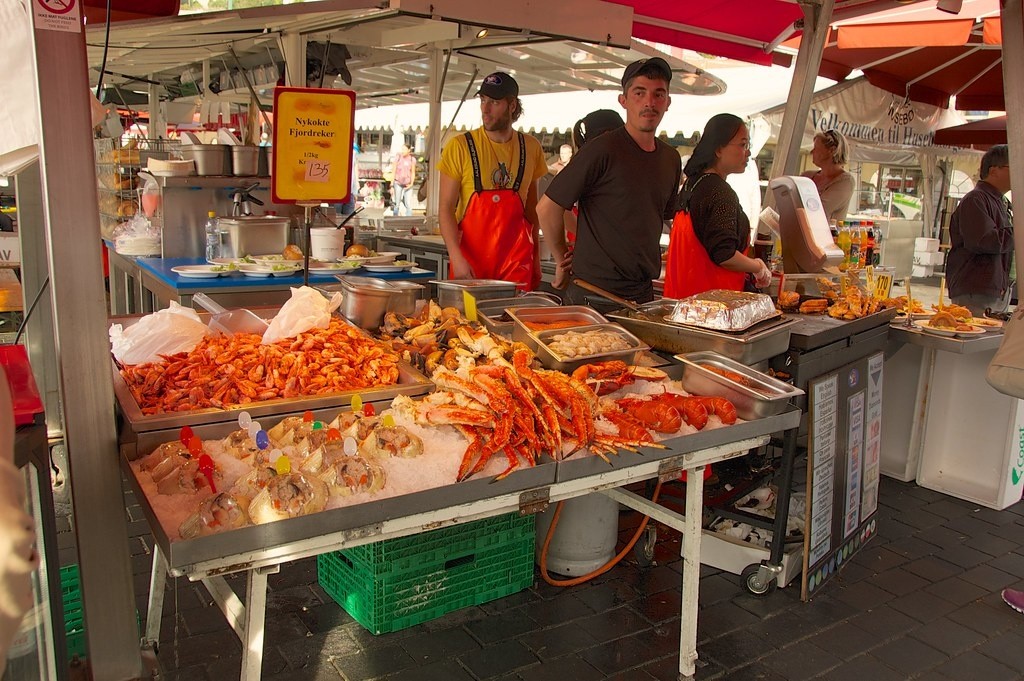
[120,317,400,416]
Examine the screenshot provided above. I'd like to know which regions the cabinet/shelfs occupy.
[411,155,427,210]
[95,136,181,243]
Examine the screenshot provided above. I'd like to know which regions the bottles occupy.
[827,219,882,271]
[205,210,222,263]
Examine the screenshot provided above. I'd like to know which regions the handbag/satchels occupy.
[984,303,1024,400]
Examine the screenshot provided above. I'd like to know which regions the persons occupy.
[535,57,682,314]
[801,130,855,221]
[330,149,358,215]
[945,144,1015,318]
[663,113,772,485]
[564,109,625,252]
[390,144,416,217]
[260,133,272,146]
[435,72,548,292]
[551,144,572,174]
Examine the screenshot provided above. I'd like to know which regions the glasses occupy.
[827,130,837,144]
[727,142,754,151]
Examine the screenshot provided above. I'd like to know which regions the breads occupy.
[282,244,303,260]
[100,138,145,240]
[345,244,369,257]
[777,291,800,307]
[799,299,828,313]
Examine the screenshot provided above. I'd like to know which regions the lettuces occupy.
[330,250,379,268]
[210,256,299,272]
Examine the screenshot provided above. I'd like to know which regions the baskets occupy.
[7,563,140,660]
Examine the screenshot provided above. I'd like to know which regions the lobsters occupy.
[614,398,682,434]
[644,392,709,430]
[600,411,654,446]
[686,396,738,426]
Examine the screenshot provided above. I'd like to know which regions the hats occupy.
[621,57,672,88]
[473,72,519,100]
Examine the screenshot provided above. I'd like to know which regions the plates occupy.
[171,252,418,278]
[888,303,1005,338]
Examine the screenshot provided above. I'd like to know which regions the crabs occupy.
[371,298,674,485]
[141,408,425,540]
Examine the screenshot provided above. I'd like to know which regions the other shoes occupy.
[1002,589,1024,614]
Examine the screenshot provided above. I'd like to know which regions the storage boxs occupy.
[879,343,1024,511]
[317,511,537,636]
[680,484,803,589]
[179,62,285,97]
[912,238,944,277]
